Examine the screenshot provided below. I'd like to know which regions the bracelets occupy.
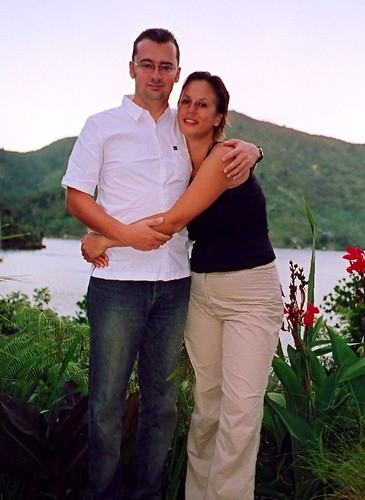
[256,145,264,163]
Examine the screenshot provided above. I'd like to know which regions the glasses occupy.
[130,59,178,75]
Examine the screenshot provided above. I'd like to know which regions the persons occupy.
[60,28,266,499]
[80,71,284,500]
